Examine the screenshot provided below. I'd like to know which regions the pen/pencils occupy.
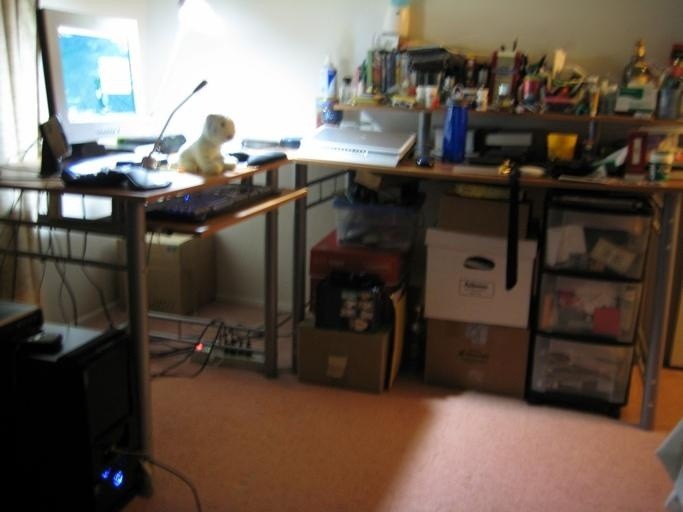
[534,53,547,76]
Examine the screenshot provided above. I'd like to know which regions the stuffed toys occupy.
[172,115,235,175]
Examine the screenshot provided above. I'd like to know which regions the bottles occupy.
[647,147,675,183]
[319,52,339,104]
[443,94,467,162]
[614,32,682,118]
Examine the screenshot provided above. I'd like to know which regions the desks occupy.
[0,138,308,462]
[290,99,682,432]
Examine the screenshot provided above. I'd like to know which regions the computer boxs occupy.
[26,321,142,510]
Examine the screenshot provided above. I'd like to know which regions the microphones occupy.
[142,78,209,170]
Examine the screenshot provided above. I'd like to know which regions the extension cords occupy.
[196,344,266,372]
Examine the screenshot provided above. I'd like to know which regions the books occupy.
[364,46,446,101]
